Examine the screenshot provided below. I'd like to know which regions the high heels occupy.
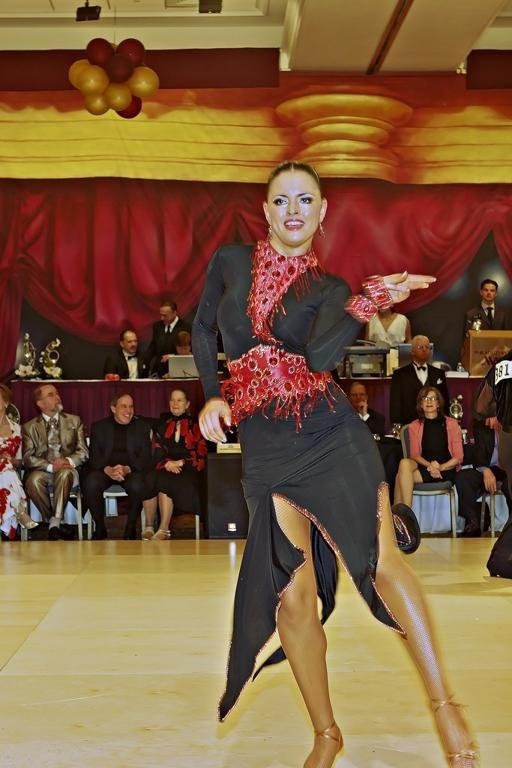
[18,511,39,530]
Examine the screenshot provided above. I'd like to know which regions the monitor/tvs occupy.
[398,343,434,367]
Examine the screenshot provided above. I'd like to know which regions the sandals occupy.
[431,695,481,768]
[142,526,154,540]
[153,529,171,540]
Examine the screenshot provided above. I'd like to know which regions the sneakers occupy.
[123,528,137,539]
[91,528,108,540]
[48,524,77,541]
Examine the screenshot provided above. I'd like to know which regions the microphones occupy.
[477,312,482,319]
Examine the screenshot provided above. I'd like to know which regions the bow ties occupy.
[128,354,136,360]
[413,361,426,371]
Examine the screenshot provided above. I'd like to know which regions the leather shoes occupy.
[457,525,481,536]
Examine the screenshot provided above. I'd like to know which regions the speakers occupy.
[207,452,249,538]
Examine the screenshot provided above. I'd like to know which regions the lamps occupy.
[75,0,102,21]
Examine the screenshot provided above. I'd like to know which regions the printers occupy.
[344,337,390,377]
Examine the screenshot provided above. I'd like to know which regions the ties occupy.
[486,307,494,324]
[166,325,171,335]
[47,419,62,459]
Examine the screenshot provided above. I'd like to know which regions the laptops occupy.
[168,355,200,378]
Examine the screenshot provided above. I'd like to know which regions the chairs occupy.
[398,421,457,538]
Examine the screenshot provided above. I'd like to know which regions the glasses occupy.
[422,396,438,401]
[416,344,430,350]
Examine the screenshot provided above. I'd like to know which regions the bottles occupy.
[457,362,463,372]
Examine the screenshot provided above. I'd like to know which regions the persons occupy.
[192,158,480,768]
[463,278,510,338]
[344,379,510,536]
[390,336,449,427]
[474,349,512,578]
[1,384,209,540]
[149,300,192,375]
[155,332,194,379]
[105,331,149,378]
[365,304,411,346]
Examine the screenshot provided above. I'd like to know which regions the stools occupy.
[480,481,505,536]
[5,476,203,540]
